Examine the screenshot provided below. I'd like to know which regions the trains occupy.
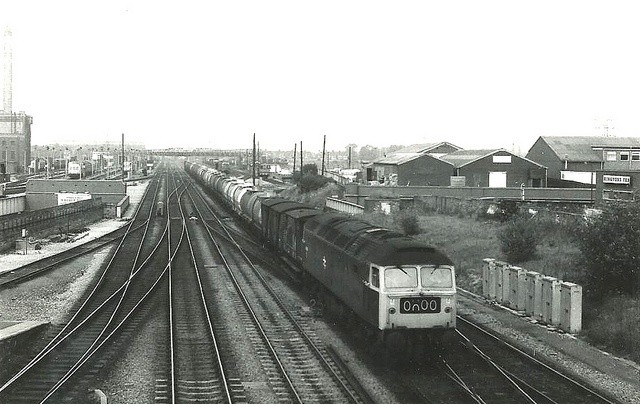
[209,159,230,171]
[184,161,456,331]
